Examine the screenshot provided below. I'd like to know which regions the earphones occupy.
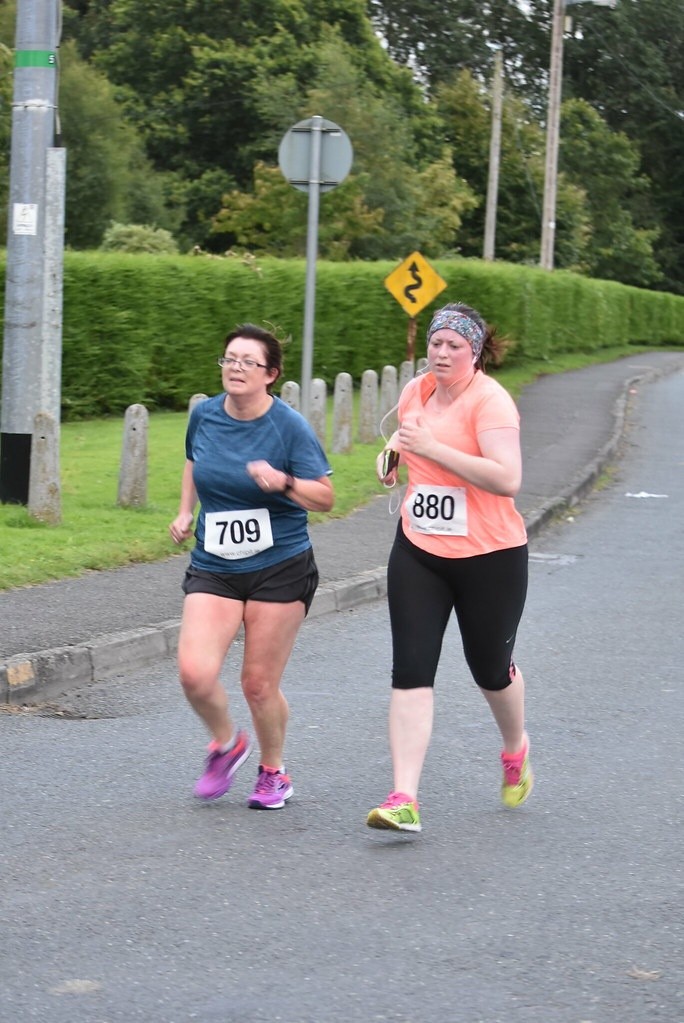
[472,356,479,365]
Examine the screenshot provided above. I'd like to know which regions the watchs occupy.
[280,472,295,495]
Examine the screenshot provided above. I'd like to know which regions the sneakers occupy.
[499,732,534,813]
[192,727,254,802]
[247,763,295,810]
[367,787,425,838]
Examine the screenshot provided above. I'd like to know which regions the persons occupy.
[367,302,536,832]
[166,318,338,812]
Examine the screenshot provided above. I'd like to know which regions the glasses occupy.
[216,355,272,374]
